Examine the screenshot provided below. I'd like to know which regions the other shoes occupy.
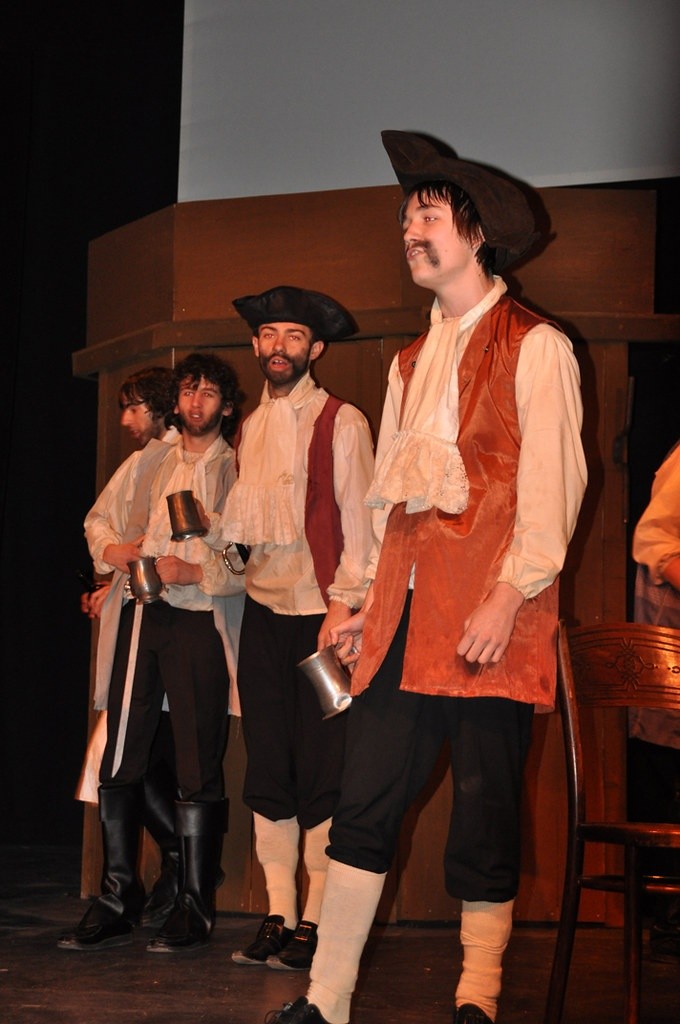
[452,1001,495,1024]
[263,994,351,1024]
[648,941,680,964]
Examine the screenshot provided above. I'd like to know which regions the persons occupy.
[632,445,680,964]
[265,132,588,1024]
[57,353,247,951]
[82,368,185,930]
[167,284,376,971]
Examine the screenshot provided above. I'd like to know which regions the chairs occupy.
[547,618,679,1024]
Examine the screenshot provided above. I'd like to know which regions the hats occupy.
[380,129,543,275]
[231,286,360,341]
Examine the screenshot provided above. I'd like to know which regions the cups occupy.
[127,556,169,604]
[297,641,359,720]
[166,490,204,541]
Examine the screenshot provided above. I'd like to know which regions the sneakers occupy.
[231,914,295,965]
[266,920,319,970]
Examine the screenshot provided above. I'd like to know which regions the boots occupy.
[56,783,149,949]
[139,775,227,925]
[145,798,231,952]
